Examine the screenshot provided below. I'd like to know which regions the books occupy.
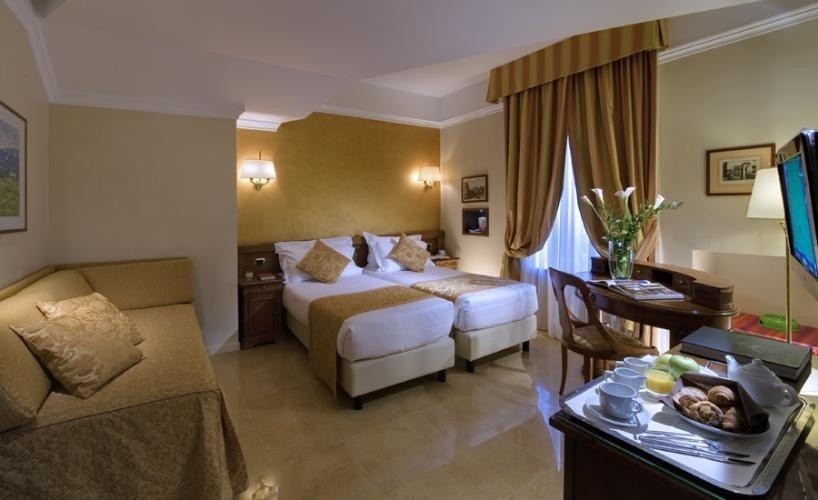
[585,276,683,301]
[681,326,812,381]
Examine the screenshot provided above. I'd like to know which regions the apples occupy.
[653,352,672,371]
[669,354,700,382]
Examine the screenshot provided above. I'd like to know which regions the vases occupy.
[607,241,635,279]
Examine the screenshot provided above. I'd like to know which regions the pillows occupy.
[0,259,196,431]
[273,236,364,283]
[363,232,434,272]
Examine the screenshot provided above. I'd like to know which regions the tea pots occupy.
[724,355,797,409]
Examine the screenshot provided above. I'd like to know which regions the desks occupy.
[574,257,740,375]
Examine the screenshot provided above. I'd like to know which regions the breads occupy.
[672,385,746,433]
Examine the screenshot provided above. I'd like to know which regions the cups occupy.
[439,250,446,256]
[612,358,651,388]
[604,368,647,394]
[597,382,643,419]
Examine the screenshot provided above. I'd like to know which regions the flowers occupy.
[582,187,684,276]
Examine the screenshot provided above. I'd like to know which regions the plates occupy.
[696,365,720,377]
[644,385,669,399]
[670,407,773,441]
[585,395,652,426]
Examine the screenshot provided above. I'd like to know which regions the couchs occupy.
[0,257,250,500]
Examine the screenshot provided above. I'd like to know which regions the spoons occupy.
[646,429,723,441]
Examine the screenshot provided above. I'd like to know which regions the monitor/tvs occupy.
[774,133,818,301]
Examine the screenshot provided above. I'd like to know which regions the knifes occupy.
[638,437,756,466]
[639,438,751,458]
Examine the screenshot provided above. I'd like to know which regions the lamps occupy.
[418,160,440,191]
[240,149,276,191]
[747,167,792,343]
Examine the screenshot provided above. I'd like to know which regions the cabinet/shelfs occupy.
[548,328,818,500]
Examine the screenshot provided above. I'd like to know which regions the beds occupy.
[362,262,539,373]
[283,274,456,410]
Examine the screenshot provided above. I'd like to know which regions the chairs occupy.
[548,267,659,396]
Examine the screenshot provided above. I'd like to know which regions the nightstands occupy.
[240,274,283,348]
[433,257,459,270]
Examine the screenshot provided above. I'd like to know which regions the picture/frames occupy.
[461,208,489,236]
[0,99,28,233]
[706,143,776,193]
[461,174,488,202]
